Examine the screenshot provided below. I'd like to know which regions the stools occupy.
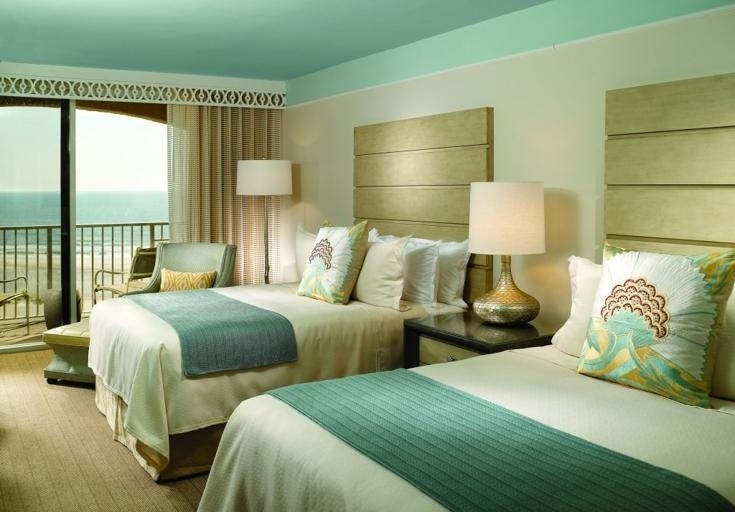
[43,287,81,330]
[42,319,95,384]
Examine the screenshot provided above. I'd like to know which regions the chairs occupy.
[117,241,237,296]
[93,246,157,305]
[0,277,30,336]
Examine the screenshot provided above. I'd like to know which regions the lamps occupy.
[235,157,294,284]
[469,180,547,326]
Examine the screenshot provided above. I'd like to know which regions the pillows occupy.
[158,268,218,292]
[294,217,473,313]
[550,240,735,410]
